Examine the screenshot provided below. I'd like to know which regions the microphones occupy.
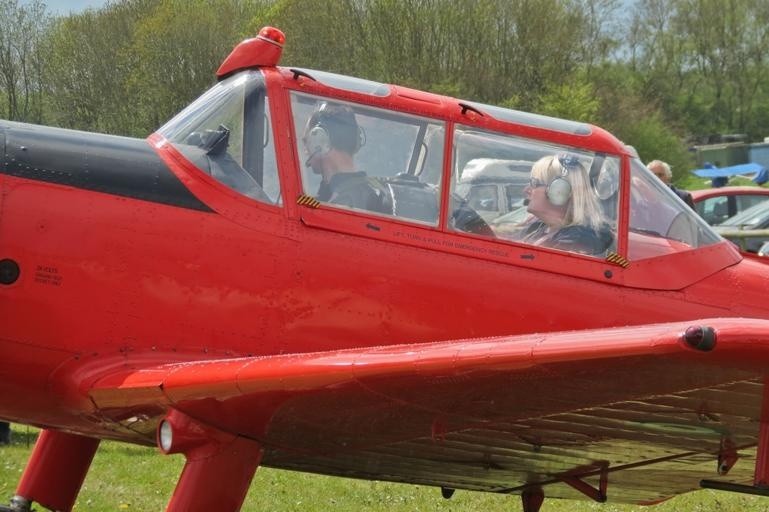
[524,199,529,206]
[306,150,318,167]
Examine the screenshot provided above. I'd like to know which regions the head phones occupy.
[307,102,367,157]
[546,151,579,206]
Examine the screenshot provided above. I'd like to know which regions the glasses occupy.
[528,178,546,188]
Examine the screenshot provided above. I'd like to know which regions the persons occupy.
[634,158,696,235]
[711,160,730,187]
[434,149,615,258]
[303,102,383,213]
